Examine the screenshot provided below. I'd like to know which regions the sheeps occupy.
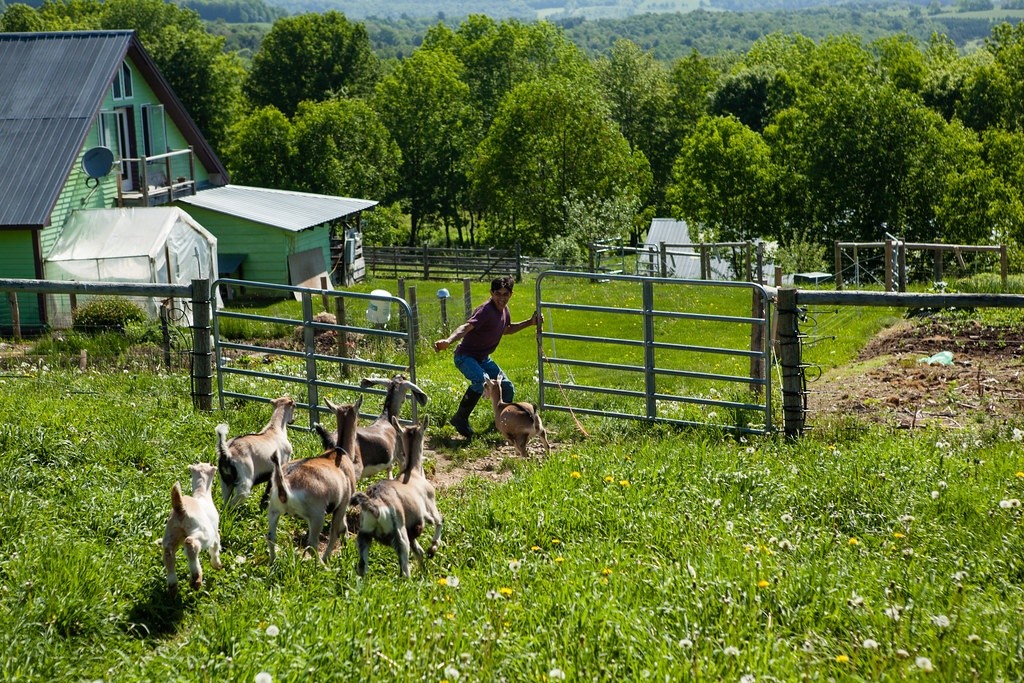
[266,393,364,568]
[347,414,444,581]
[313,375,429,494]
[480,371,551,458]
[215,396,294,507]
[161,462,222,595]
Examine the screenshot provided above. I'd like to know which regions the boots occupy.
[450,385,483,437]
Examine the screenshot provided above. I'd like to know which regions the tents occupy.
[45,205,227,325]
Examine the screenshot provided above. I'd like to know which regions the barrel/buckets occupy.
[366,289,390,324]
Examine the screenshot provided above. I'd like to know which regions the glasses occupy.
[492,291,511,297]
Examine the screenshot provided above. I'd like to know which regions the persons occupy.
[434,277,546,436]
